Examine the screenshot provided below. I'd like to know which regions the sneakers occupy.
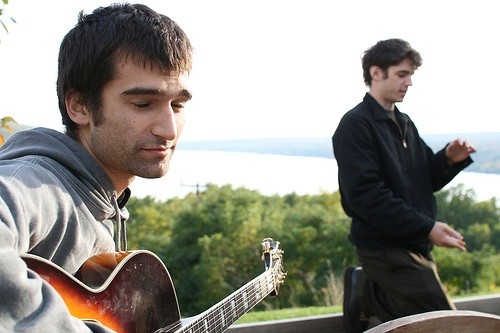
[344,265,370,333]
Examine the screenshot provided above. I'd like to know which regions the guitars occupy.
[18,237,289,333]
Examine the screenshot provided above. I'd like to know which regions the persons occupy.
[332,38,478,333]
[0,2,192,333]
